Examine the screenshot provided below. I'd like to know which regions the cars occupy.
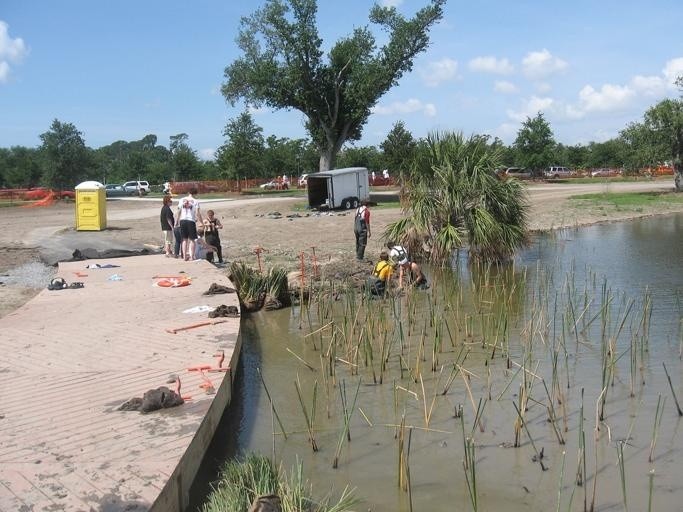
[590,168,616,177]
[53,188,75,201]
[259,179,290,191]
[296,174,307,188]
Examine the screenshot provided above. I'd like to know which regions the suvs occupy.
[103,180,150,194]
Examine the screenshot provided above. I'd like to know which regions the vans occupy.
[542,166,579,178]
[504,165,535,179]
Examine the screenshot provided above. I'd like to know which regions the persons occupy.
[370,252,391,300]
[395,253,427,292]
[157,192,226,265]
[275,174,282,190]
[282,172,288,190]
[353,201,371,260]
[385,241,408,269]
